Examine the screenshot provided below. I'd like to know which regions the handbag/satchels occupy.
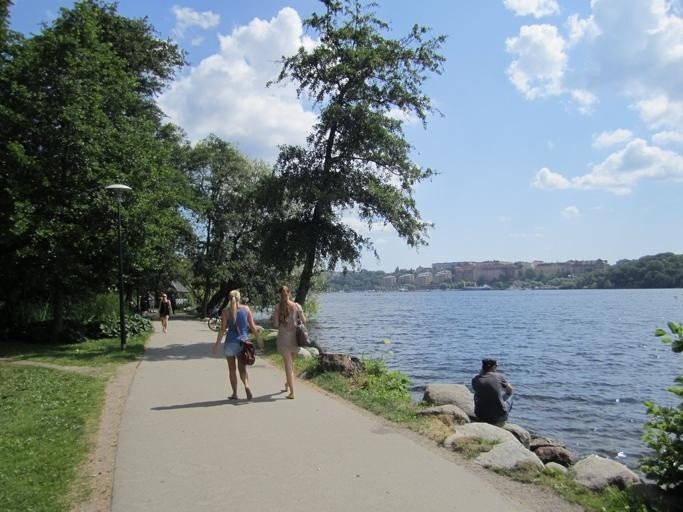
[241,339,255,365]
[296,321,311,347]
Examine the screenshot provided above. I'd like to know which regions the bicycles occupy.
[208,304,228,335]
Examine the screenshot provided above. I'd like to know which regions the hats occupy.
[482,359,497,366]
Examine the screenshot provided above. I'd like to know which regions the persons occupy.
[137,290,176,317]
[472,357,513,422]
[271,284,306,400]
[209,289,263,400]
[157,294,173,334]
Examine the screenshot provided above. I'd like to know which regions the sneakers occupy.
[285,394,295,400]
[228,395,238,400]
[245,385,252,401]
[285,382,289,392]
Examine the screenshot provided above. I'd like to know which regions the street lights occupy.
[106,183,134,350]
